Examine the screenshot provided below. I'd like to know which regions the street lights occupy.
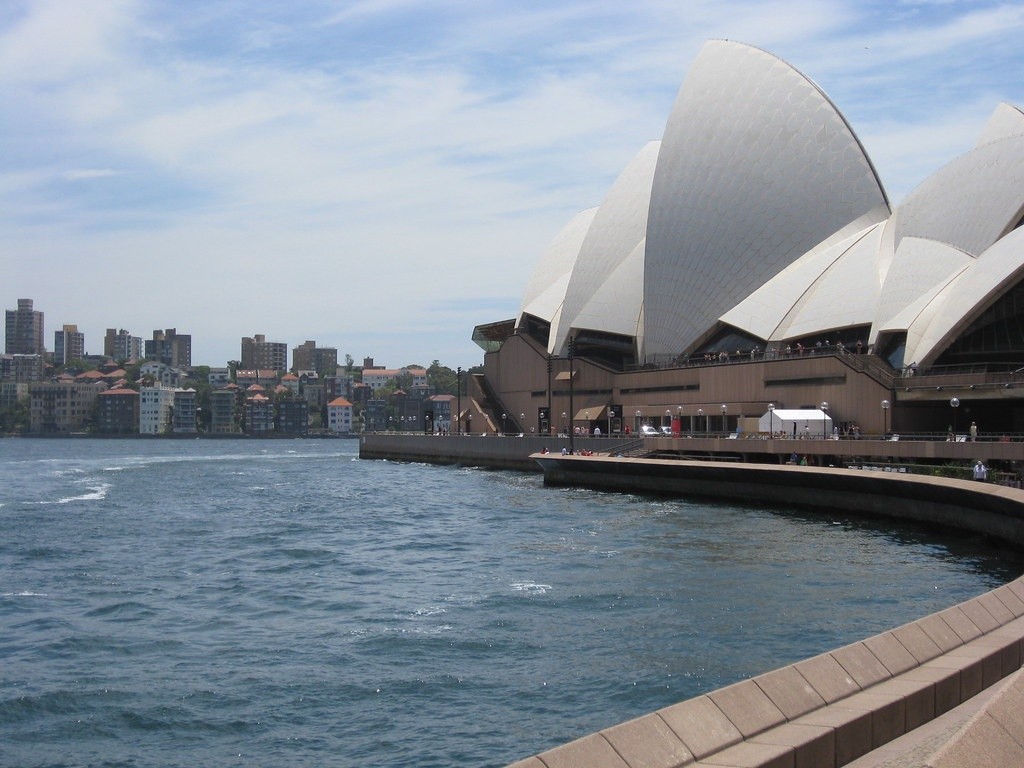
[456,367,462,436]
[821,401,829,440]
[721,405,727,438]
[610,411,615,437]
[501,413,506,437]
[540,413,544,436]
[697,408,703,438]
[677,406,682,439]
[546,353,555,436]
[568,336,575,456]
[882,400,889,440]
[521,413,525,437]
[768,404,776,439]
[562,412,566,438]
[636,410,641,438]
[439,415,444,436]
[951,398,959,442]
[485,414,489,436]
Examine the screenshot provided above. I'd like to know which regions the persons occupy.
[527,425,630,457]
[436,424,452,436]
[773,422,987,482]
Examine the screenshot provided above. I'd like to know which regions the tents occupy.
[759,409,832,441]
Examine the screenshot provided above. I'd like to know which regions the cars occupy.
[640,427,660,437]
[660,427,672,438]
